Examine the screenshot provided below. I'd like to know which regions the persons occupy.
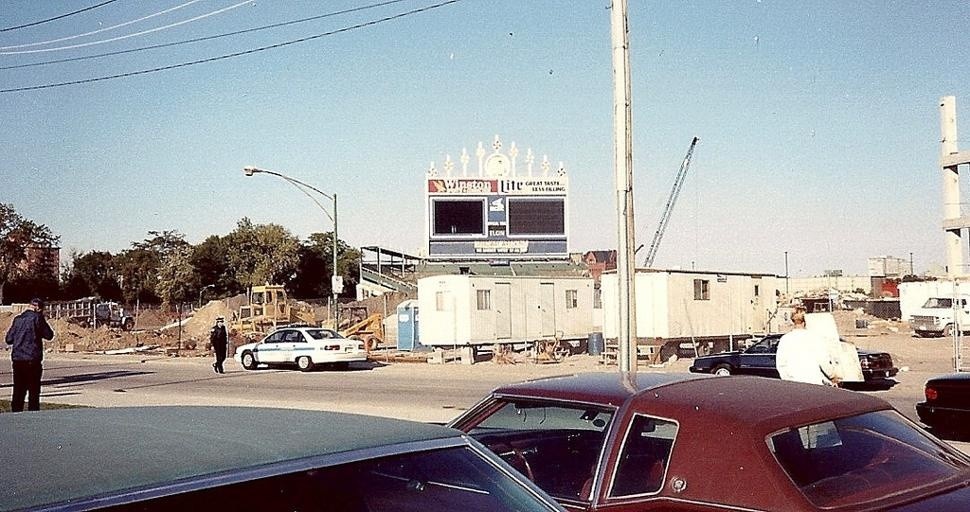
[5,296,55,413]
[209,315,230,374]
[773,312,840,451]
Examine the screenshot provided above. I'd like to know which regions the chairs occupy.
[578,451,664,501]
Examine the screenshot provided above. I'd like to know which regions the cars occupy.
[911,295,969,336]
[233,325,368,371]
[693,332,894,386]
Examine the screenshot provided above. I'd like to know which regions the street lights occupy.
[243,164,337,339]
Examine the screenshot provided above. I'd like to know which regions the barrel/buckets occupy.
[588,331,604,356]
